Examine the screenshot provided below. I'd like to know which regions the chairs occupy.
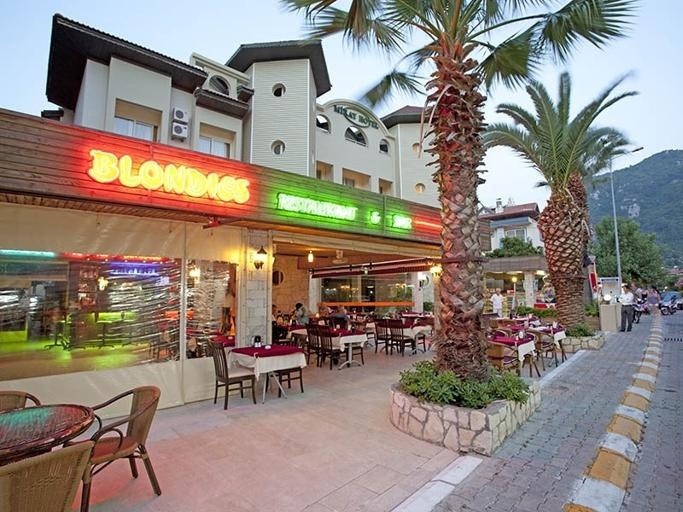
[60,380,163,511]
[159,323,233,357]
[264,333,309,398]
[1,389,54,463]
[481,312,570,380]
[205,338,258,410]
[1,438,96,511]
[273,309,434,370]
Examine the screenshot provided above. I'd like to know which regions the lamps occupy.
[253,246,267,271]
[307,247,314,264]
[417,272,429,291]
[428,259,441,278]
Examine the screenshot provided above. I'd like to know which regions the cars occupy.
[659,290,683,310]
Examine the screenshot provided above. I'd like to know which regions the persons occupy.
[229,291,235,332]
[490,287,504,319]
[318,301,330,311]
[47,298,67,345]
[289,302,309,325]
[330,303,352,330]
[271,303,277,322]
[617,281,660,331]
[221,287,230,329]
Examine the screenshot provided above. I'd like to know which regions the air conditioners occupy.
[169,121,188,141]
[169,107,189,125]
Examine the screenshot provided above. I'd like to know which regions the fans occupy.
[330,249,348,265]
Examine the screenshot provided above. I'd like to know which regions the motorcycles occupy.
[630,297,647,324]
[660,300,679,316]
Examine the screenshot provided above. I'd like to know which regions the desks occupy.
[224,343,307,403]
[0,403,96,462]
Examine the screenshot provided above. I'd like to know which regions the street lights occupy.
[608,146,645,294]
[510,276,518,312]
